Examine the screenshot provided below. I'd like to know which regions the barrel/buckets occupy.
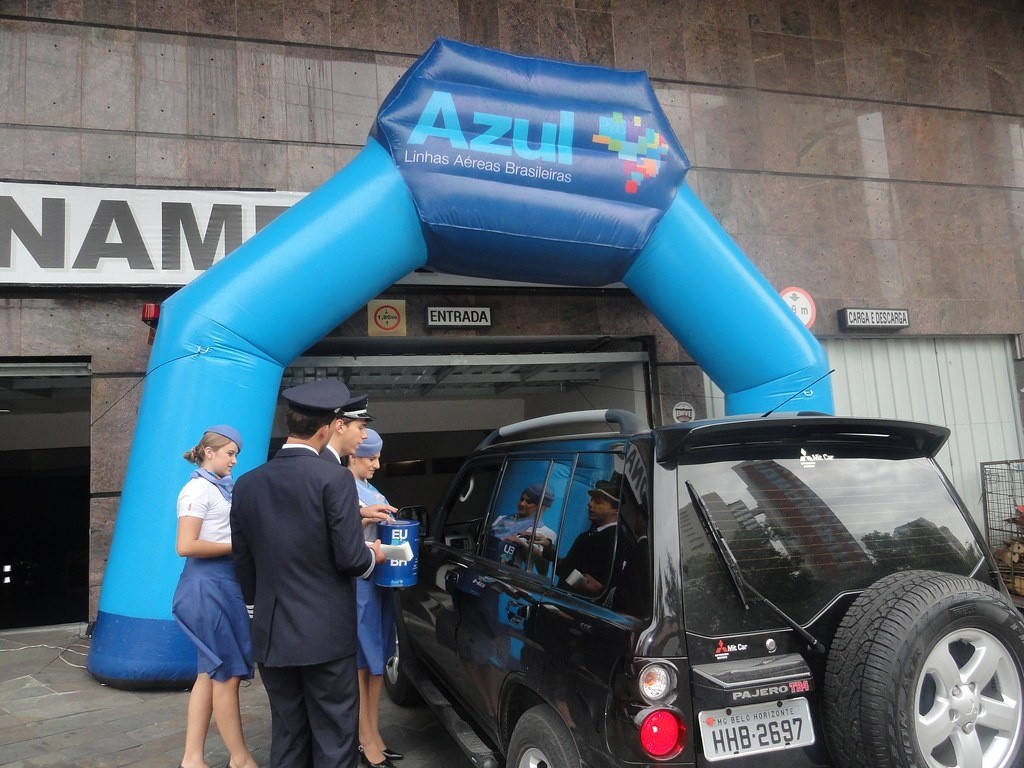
[376,516,418,590]
[456,530,515,599]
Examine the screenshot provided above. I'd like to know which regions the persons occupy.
[444,481,557,728]
[176,424,263,768]
[228,375,388,767]
[346,426,403,768]
[320,391,399,523]
[511,480,631,736]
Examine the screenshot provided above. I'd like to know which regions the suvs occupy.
[380,405,1022,768]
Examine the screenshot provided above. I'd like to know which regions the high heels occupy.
[358,740,397,768]
[381,749,403,761]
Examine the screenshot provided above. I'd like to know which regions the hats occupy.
[335,393,377,422]
[625,488,648,518]
[204,425,242,454]
[587,480,627,507]
[354,427,383,457]
[281,379,351,419]
[526,484,555,506]
